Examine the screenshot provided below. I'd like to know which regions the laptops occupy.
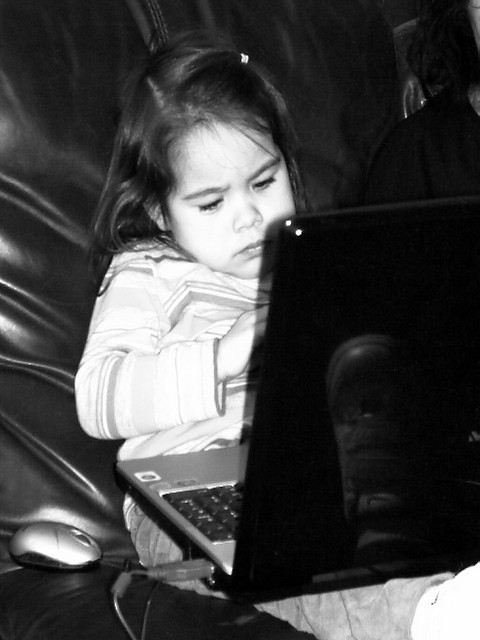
[115,196,480,604]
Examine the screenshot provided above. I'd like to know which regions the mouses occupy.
[6,520,105,571]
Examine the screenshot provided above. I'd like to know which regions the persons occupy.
[360,0,478,202]
[75,30,479,640]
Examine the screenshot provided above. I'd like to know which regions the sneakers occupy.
[328,336,430,522]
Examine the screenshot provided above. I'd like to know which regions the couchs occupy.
[0,0,480,640]
